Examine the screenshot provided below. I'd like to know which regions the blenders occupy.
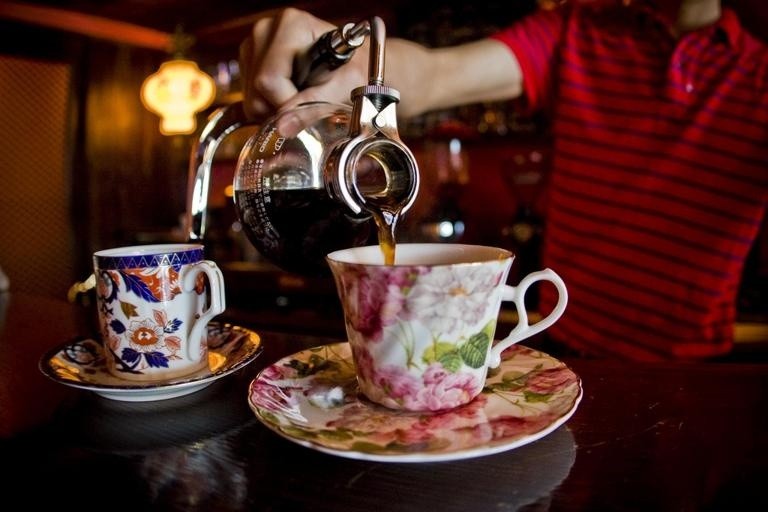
[498,152,550,241]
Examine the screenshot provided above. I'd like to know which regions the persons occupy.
[236,0,766,368]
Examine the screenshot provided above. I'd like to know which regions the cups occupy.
[328,243,571,411]
[95,243,227,383]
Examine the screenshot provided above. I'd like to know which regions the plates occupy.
[245,335,590,465]
[43,325,264,415]
[62,377,258,456]
[298,423,581,512]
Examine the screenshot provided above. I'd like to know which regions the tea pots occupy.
[232,17,421,282]
